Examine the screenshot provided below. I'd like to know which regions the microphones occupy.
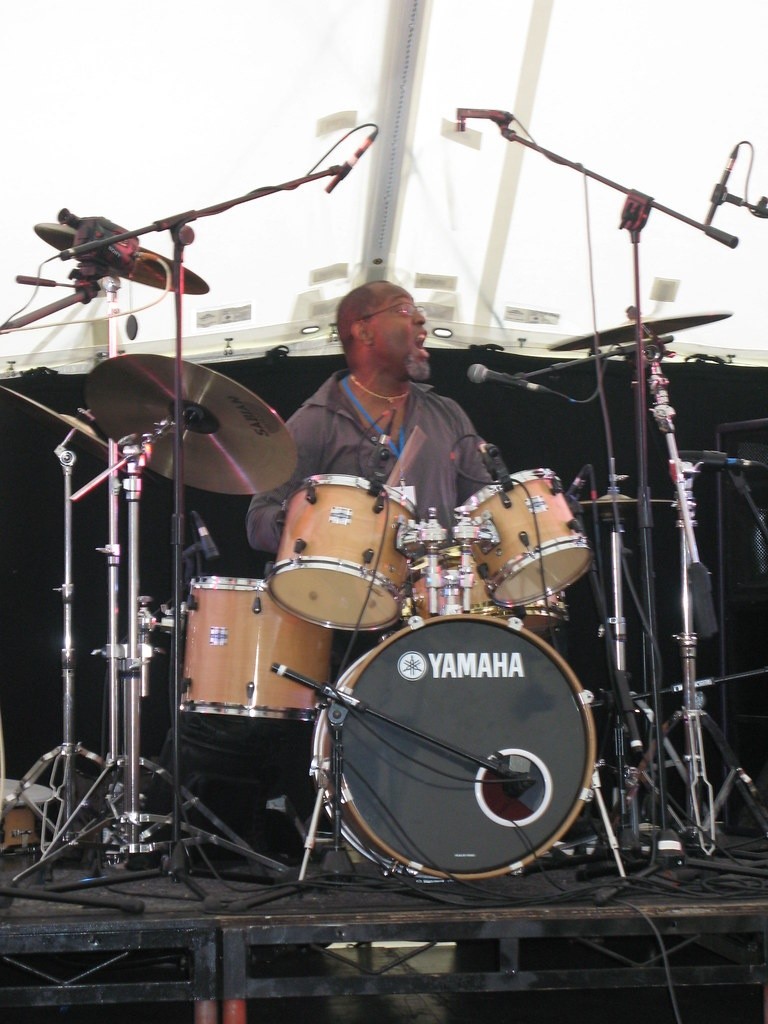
[457,108,515,122]
[324,129,378,194]
[367,410,396,467]
[501,771,536,799]
[468,364,550,392]
[706,144,740,225]
[473,438,498,483]
[565,465,591,497]
[678,450,767,471]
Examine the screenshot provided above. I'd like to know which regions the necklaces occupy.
[350,372,410,404]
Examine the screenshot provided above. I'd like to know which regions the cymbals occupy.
[83,354,297,495]
[549,311,734,352]
[579,492,675,518]
[33,222,211,296]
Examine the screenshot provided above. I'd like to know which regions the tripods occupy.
[497,120,768,907]
[0,166,513,919]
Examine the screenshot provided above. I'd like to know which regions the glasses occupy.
[358,303,425,321]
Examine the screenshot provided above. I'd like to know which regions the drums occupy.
[404,544,568,637]
[179,575,332,723]
[457,468,591,608]
[309,616,597,878]
[267,473,416,632]
[0,778,53,856]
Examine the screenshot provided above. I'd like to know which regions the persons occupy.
[244,281,499,680]
